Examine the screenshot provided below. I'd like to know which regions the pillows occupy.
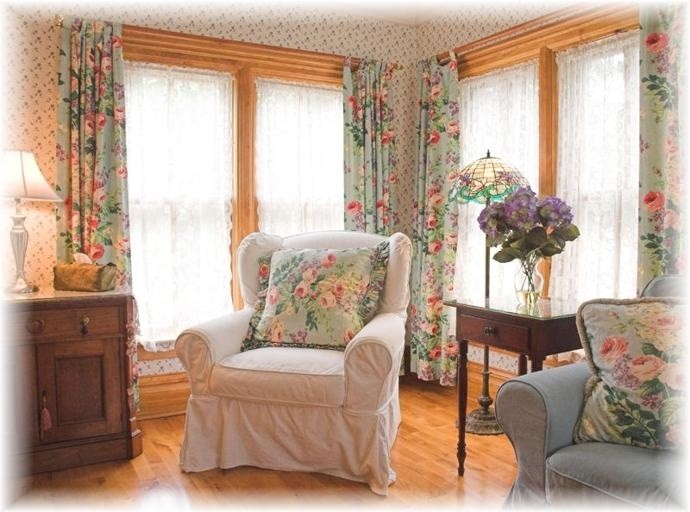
[239,238,391,353]
[571,297,685,452]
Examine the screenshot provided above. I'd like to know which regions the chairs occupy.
[173,230,414,484]
[493,272,685,509]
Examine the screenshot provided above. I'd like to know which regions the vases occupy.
[513,254,545,305]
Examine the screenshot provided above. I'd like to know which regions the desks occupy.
[442,296,583,476]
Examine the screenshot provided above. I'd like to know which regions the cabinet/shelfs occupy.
[3,285,144,478]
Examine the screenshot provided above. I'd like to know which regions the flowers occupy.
[476,185,581,303]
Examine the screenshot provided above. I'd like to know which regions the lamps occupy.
[446,146,534,436]
[0,146,64,295]
